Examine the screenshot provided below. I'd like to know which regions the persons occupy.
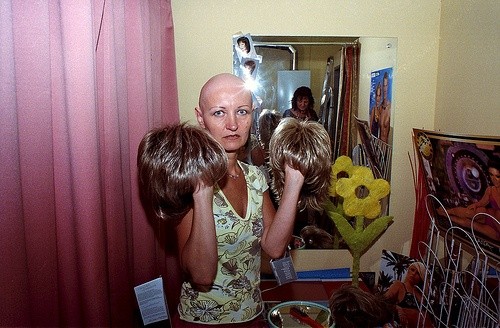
[283,86,319,122]
[436,161,500,241]
[174,72,305,328]
[237,37,251,54]
[369,82,382,140]
[243,60,256,75]
[381,262,436,328]
[379,72,392,144]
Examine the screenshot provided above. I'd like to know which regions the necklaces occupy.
[227,169,242,179]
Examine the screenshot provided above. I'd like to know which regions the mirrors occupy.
[232,36,398,251]
